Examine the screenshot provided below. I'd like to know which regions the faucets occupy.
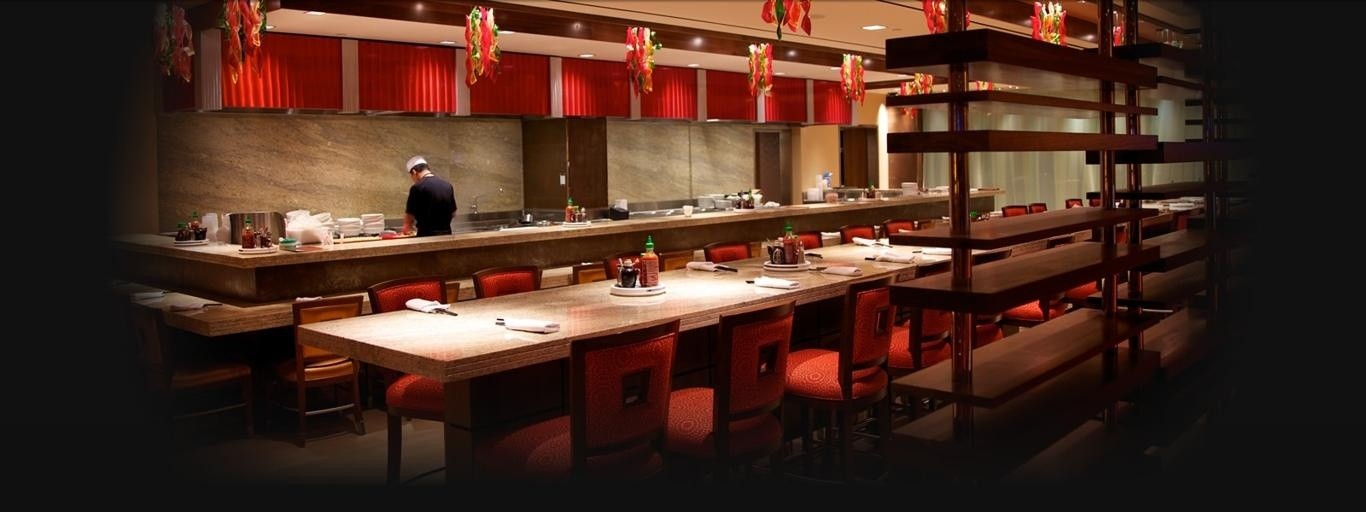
[471,187,504,205]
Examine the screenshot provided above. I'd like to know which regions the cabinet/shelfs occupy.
[1083,1,1229,489]
[887,2,1158,489]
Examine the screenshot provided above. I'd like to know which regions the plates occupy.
[697,194,733,209]
[763,261,812,271]
[335,211,386,237]
[610,285,670,296]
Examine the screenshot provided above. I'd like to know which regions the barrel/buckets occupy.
[901,181,918,197]
[820,231,841,247]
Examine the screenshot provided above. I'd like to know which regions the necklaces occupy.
[423,172,433,176]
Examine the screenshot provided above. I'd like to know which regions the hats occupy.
[405,154,428,173]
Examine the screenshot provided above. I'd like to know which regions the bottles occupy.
[574,206,587,223]
[257,229,272,247]
[640,235,659,288]
[736,187,754,209]
[565,197,575,223]
[240,216,255,249]
[784,225,800,263]
[616,258,637,288]
[971,211,977,222]
[175,211,207,240]
[766,237,785,264]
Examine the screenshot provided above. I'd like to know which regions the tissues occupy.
[608,198,629,219]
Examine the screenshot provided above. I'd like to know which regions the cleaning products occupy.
[824,170,834,191]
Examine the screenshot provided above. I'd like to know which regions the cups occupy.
[202,212,218,232]
[320,231,333,249]
[682,205,693,217]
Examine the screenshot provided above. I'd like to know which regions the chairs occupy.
[114,197,1191,489]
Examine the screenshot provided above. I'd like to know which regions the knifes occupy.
[434,306,459,318]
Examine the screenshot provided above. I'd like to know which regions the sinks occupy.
[453,219,519,233]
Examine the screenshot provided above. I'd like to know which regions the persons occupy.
[402,155,458,236]
